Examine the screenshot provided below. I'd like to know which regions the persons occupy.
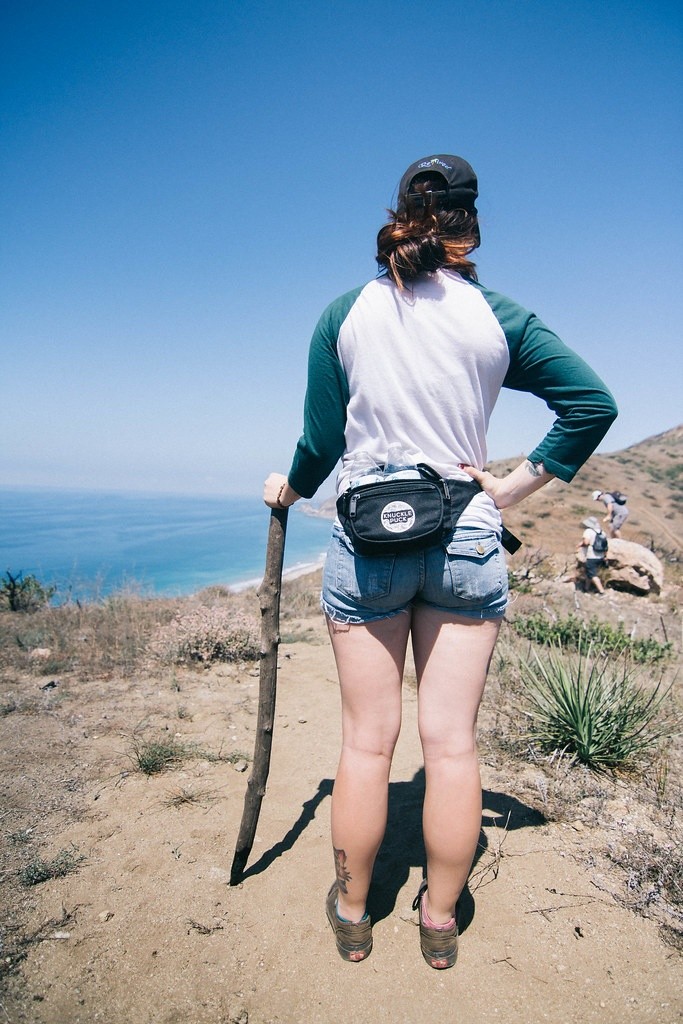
[592,490,629,539]
[263,154,618,969]
[575,517,607,600]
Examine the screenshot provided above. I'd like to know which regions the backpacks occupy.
[604,490,627,505]
[591,528,608,551]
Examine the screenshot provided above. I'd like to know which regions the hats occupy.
[582,517,601,529]
[592,490,602,500]
[397,154,478,206]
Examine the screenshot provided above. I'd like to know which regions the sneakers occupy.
[412,878,458,969]
[326,879,372,962]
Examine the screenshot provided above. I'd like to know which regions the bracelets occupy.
[276,483,294,508]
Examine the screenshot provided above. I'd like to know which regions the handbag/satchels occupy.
[336,462,483,548]
